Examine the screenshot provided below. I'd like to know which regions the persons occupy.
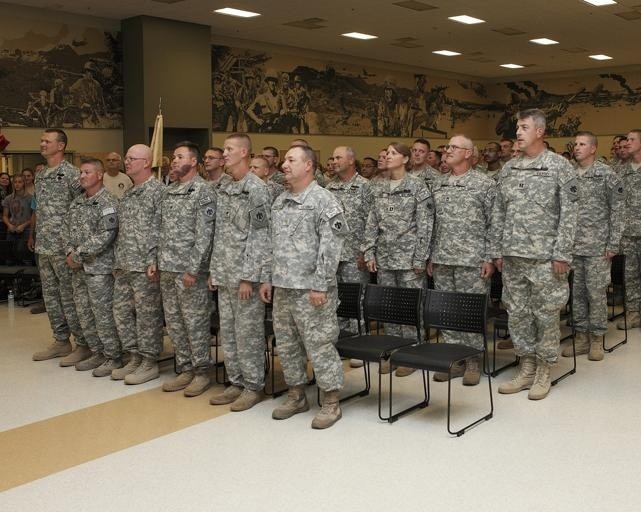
[374,79,449,134]
[493,92,522,141]
[212,71,311,135]
[25,61,121,117]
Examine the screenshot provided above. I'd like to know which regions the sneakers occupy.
[125,356,159,384]
[209,385,243,406]
[462,358,482,385]
[349,358,364,368]
[615,312,640,331]
[379,358,419,377]
[271,385,309,420]
[562,333,590,359]
[311,389,342,429]
[31,300,46,315]
[498,357,535,395]
[182,373,211,397]
[527,357,552,400]
[433,359,465,382]
[110,355,138,382]
[161,372,192,393]
[33,339,72,361]
[587,331,606,361]
[59,346,123,377]
[230,389,265,411]
[497,338,515,350]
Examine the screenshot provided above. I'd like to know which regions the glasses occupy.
[445,144,470,151]
[202,156,224,161]
[124,157,147,163]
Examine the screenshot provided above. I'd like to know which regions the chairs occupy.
[335,283,427,422]
[272,283,368,407]
[0,239,40,308]
[370,255,627,389]
[157,287,316,395]
[390,289,493,437]
